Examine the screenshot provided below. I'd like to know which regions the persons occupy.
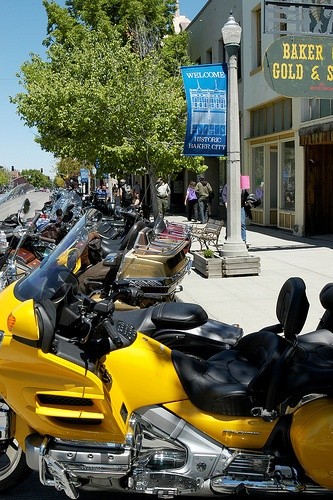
[96,179,142,210]
[184,181,198,223]
[155,178,171,219]
[221,173,252,248]
[195,176,212,224]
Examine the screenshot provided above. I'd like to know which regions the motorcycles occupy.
[0,207,333,496]
[1,184,244,350]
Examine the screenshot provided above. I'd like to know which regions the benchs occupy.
[189,217,224,252]
